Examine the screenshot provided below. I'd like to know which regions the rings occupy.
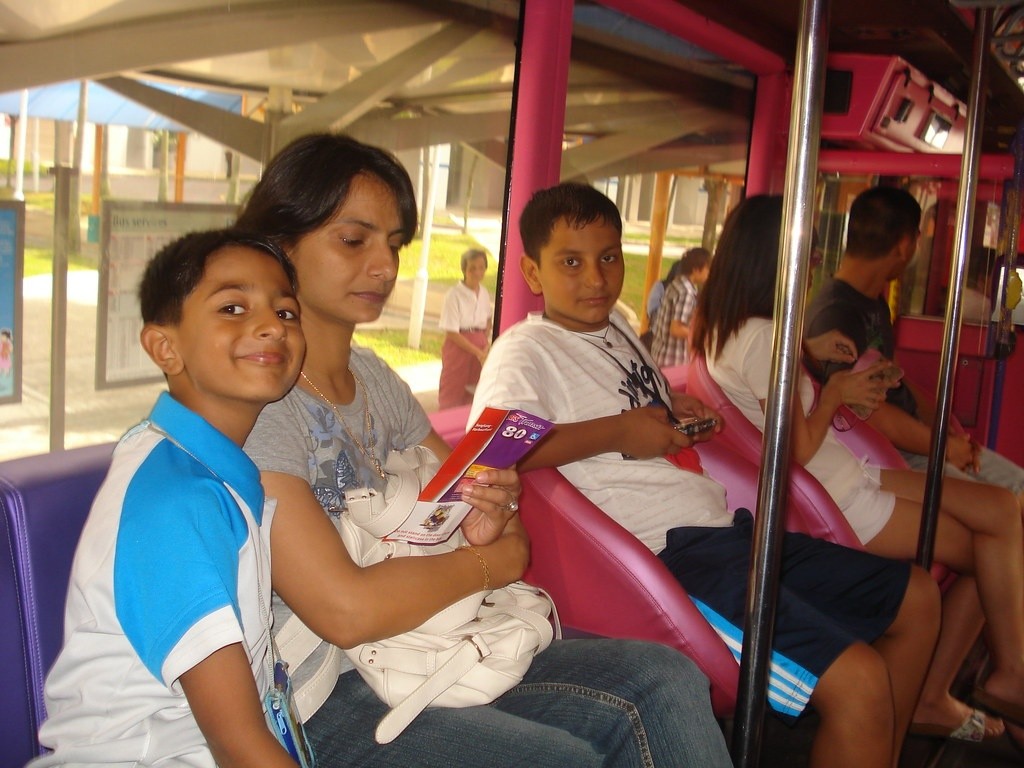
[502,493,519,511]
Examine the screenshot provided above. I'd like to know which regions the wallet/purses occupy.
[842,347,905,421]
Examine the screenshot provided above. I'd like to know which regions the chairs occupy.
[684,347,946,581]
[0,440,123,768]
[517,437,809,718]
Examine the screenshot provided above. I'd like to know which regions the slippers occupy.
[908,703,1005,747]
[973,685,1024,721]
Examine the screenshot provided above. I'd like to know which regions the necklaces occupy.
[299,362,389,482]
[542,312,614,349]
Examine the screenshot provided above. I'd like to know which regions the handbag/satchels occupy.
[277,444,561,745]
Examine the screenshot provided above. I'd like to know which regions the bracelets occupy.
[455,545,491,592]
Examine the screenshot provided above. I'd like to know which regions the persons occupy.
[226,128,736,767]
[936,244,1024,329]
[803,183,1023,502]
[23,225,311,768]
[437,248,493,412]
[638,245,712,368]
[686,193,1024,746]
[463,178,944,768]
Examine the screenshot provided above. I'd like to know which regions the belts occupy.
[460,328,487,332]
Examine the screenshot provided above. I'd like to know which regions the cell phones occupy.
[622,419,717,460]
